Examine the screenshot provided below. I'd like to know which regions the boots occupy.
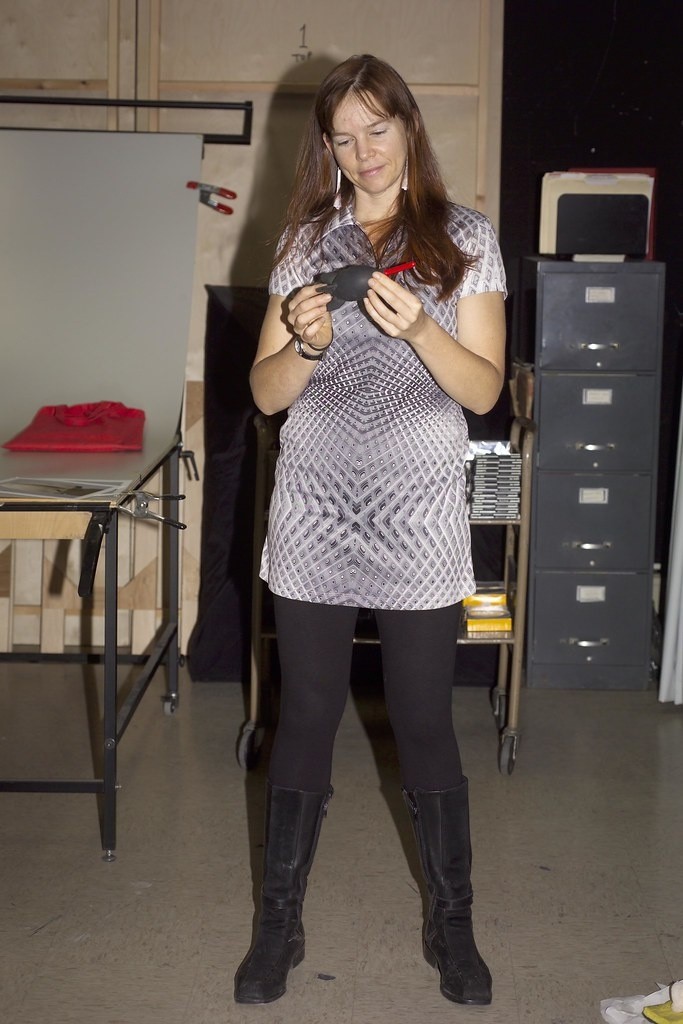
[402,775,492,1005]
[234,777,334,1002]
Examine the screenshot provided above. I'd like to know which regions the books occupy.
[571,253,627,263]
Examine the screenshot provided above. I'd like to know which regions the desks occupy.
[1,433,184,859]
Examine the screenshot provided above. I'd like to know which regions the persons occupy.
[234,55,509,1005]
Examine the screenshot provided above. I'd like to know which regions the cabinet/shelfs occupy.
[514,251,665,692]
[238,416,535,779]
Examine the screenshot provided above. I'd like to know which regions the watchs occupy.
[294,335,323,360]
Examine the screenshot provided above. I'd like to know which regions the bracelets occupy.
[308,329,333,351]
